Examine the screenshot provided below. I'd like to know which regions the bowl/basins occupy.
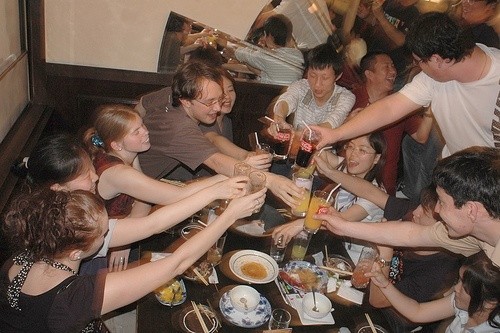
[231,284,260,313]
[229,249,279,284]
[302,291,332,319]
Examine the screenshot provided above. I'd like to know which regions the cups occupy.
[207,122,378,330]
[208,28,224,55]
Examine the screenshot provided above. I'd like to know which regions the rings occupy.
[119,256,124,263]
[256,209,260,213]
[256,199,260,204]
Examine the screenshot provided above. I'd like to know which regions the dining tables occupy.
[136,163,394,333]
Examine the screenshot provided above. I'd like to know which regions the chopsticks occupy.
[365,313,377,333]
[191,300,209,333]
[318,265,353,275]
[191,265,209,286]
[160,178,186,188]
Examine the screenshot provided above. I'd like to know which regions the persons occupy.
[0,0,500,333]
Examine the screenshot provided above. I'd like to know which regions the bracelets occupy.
[423,113,433,117]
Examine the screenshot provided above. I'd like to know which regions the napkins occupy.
[337,283,364,304]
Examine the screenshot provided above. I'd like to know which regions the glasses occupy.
[412,59,424,66]
[461,0,486,6]
[192,95,227,107]
[343,142,379,156]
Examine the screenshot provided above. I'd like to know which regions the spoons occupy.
[312,287,319,312]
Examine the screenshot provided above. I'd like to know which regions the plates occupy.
[183,262,213,280]
[323,253,355,280]
[219,289,272,328]
[178,305,220,333]
[224,62,255,75]
[231,217,272,237]
[293,297,336,326]
[355,323,389,333]
[282,260,328,293]
[155,278,186,306]
